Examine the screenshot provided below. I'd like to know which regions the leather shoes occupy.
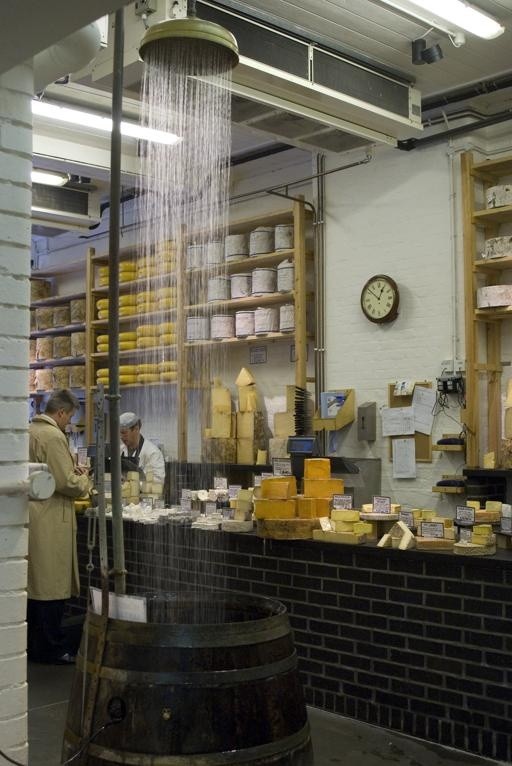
[47,653,75,665]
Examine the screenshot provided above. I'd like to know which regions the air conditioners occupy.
[30,184,103,238]
[180,0,425,150]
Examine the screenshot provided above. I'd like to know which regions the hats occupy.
[119,412,139,429]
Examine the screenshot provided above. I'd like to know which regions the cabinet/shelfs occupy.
[30,256,87,396]
[430,433,468,495]
[180,197,315,458]
[457,156,512,485]
[85,225,185,451]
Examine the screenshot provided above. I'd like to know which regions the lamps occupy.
[33,97,184,147]
[31,169,72,188]
[400,0,507,41]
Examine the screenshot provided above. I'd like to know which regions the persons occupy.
[115,412,167,492]
[31,390,93,667]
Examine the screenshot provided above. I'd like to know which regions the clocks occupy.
[357,273,400,324]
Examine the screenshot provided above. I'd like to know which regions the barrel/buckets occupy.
[61,590,313,765]
